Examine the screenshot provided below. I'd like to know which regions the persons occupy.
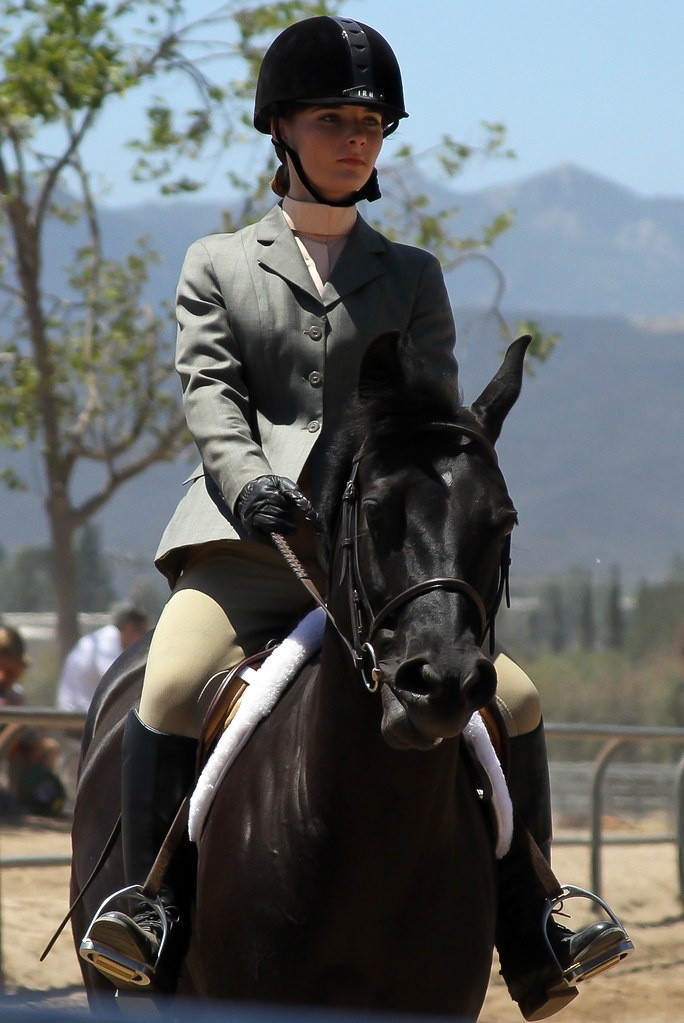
[81,18,633,1022]
[1,625,68,827]
[51,601,151,718]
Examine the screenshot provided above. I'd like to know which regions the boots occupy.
[486,717,624,1021]
[89,708,212,1023]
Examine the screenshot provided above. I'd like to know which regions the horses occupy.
[69,333,538,1023]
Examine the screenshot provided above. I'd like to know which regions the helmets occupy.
[253,14,409,135]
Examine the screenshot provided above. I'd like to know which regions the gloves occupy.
[237,475,323,543]
